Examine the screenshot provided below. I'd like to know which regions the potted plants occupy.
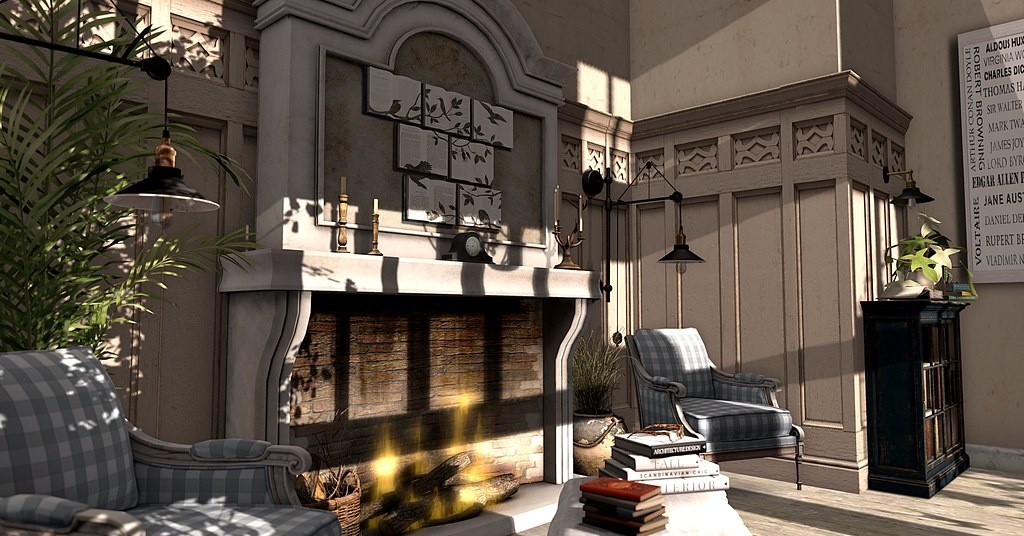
[884,212,980,297]
[571,326,626,478]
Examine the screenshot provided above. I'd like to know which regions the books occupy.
[578,430,730,536]
[402,174,456,225]
[396,123,448,176]
[876,284,977,301]
[922,323,961,460]
[457,183,502,232]
[422,83,470,137]
[447,136,494,186]
[471,99,513,148]
[365,67,422,125]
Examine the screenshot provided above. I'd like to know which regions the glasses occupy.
[627,423,684,443]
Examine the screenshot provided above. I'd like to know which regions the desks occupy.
[547,477,754,536]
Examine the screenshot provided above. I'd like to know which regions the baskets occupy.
[298,469,360,536]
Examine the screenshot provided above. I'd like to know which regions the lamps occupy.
[883,165,935,209]
[0,30,222,230]
[601,160,706,302]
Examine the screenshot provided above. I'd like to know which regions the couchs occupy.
[624,327,806,492]
[1,345,346,536]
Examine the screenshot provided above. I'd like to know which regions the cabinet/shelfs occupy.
[857,298,970,500]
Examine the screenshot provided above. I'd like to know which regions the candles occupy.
[554,188,557,225]
[373,198,379,214]
[557,185,559,220]
[341,176,346,195]
[580,195,582,231]
